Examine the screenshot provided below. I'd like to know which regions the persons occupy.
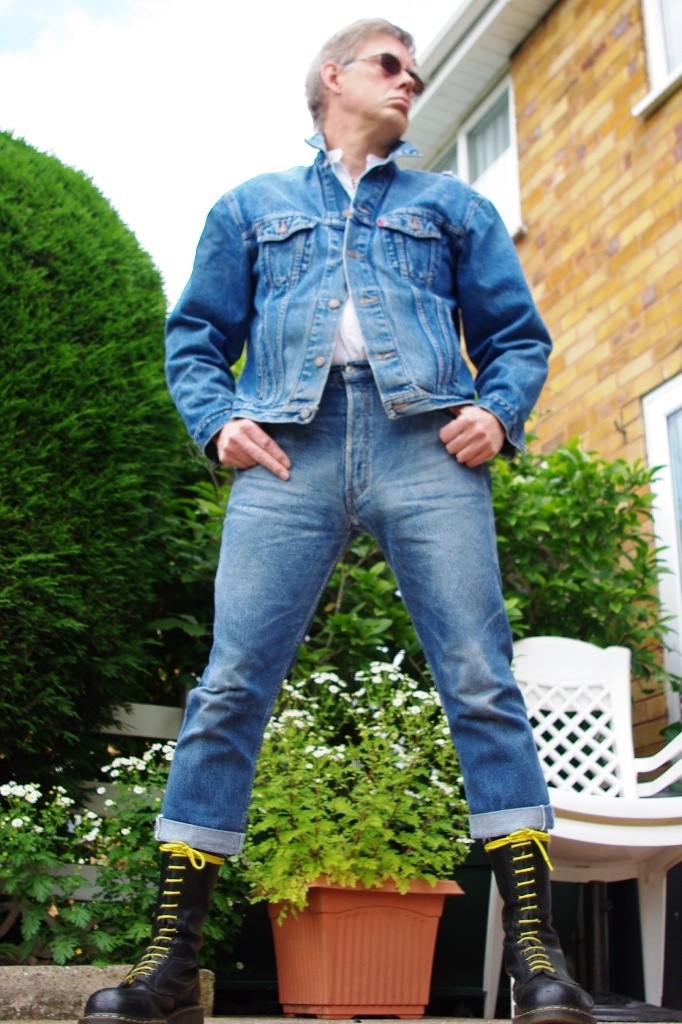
[83,17,595,1024]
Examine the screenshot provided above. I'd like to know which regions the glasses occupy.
[338,54,425,98]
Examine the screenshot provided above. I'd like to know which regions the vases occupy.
[253,874,464,1020]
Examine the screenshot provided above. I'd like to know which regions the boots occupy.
[480,827,591,1024]
[79,841,224,1024]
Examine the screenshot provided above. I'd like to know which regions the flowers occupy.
[229,651,477,905]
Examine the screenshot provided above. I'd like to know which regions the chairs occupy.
[483,636,682,1020]
